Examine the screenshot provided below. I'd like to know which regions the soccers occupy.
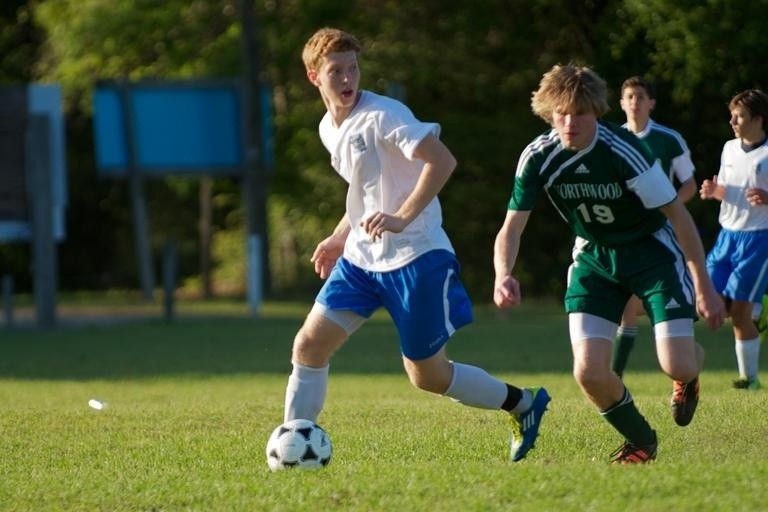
[266,419,331,472]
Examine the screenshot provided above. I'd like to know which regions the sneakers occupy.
[511,386,551,463]
[669,377,700,426]
[732,377,762,391]
[610,430,658,465]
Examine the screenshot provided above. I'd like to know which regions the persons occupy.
[284,25,555,466]
[695,86,768,392]
[610,73,701,381]
[491,59,728,463]
